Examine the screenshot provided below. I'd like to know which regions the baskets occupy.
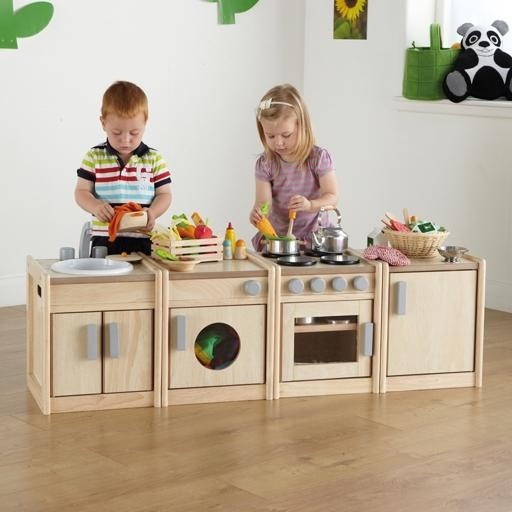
[381,228,449,259]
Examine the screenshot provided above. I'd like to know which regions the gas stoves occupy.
[256,246,370,274]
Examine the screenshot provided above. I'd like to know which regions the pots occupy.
[260,236,306,255]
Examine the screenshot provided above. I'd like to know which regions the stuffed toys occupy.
[444,19,512,104]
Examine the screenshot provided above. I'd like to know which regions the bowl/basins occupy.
[164,255,202,270]
[436,246,469,264]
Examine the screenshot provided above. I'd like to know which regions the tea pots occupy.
[310,205,348,252]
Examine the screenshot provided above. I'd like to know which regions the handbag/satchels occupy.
[403,23,460,102]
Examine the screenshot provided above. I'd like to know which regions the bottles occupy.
[223,240,233,260]
[226,221,235,256]
[235,240,248,260]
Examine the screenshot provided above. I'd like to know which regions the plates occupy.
[106,254,142,261]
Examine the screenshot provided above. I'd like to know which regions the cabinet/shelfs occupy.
[137,251,276,407]
[246,249,383,401]
[26,252,161,416]
[351,251,486,394]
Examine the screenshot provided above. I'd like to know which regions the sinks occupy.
[52,256,135,275]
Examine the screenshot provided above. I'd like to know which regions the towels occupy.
[108,202,142,242]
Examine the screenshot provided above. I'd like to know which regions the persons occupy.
[73,80,172,258]
[249,83,340,253]
